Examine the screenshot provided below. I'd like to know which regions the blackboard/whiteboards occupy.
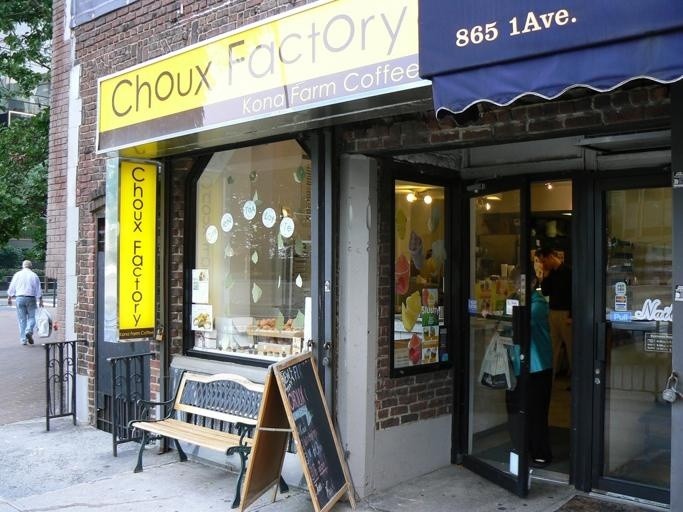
[275,352,348,511]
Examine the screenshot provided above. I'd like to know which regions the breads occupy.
[256,318,293,332]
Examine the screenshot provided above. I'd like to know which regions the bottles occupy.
[479,280,492,312]
[631,276,639,286]
[422,306,443,364]
[218,341,287,358]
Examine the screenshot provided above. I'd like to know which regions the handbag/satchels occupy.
[35,304,52,338]
[477,332,518,390]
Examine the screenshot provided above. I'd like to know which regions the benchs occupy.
[127,371,289,509]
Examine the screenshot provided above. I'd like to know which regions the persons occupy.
[5,260,44,345]
[495,265,555,467]
[532,247,573,391]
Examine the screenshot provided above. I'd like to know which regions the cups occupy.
[408,342,421,365]
[394,217,408,240]
[408,239,426,271]
[425,215,439,234]
[500,263,515,281]
[424,252,444,273]
[395,264,410,295]
[402,306,419,334]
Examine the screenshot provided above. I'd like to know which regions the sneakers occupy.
[21,333,35,345]
[530,456,552,469]
